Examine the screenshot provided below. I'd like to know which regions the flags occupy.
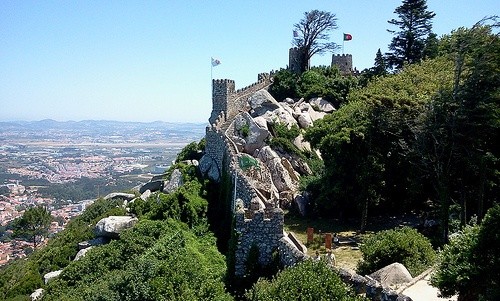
[212,57,223,67]
[344,34,352,41]
